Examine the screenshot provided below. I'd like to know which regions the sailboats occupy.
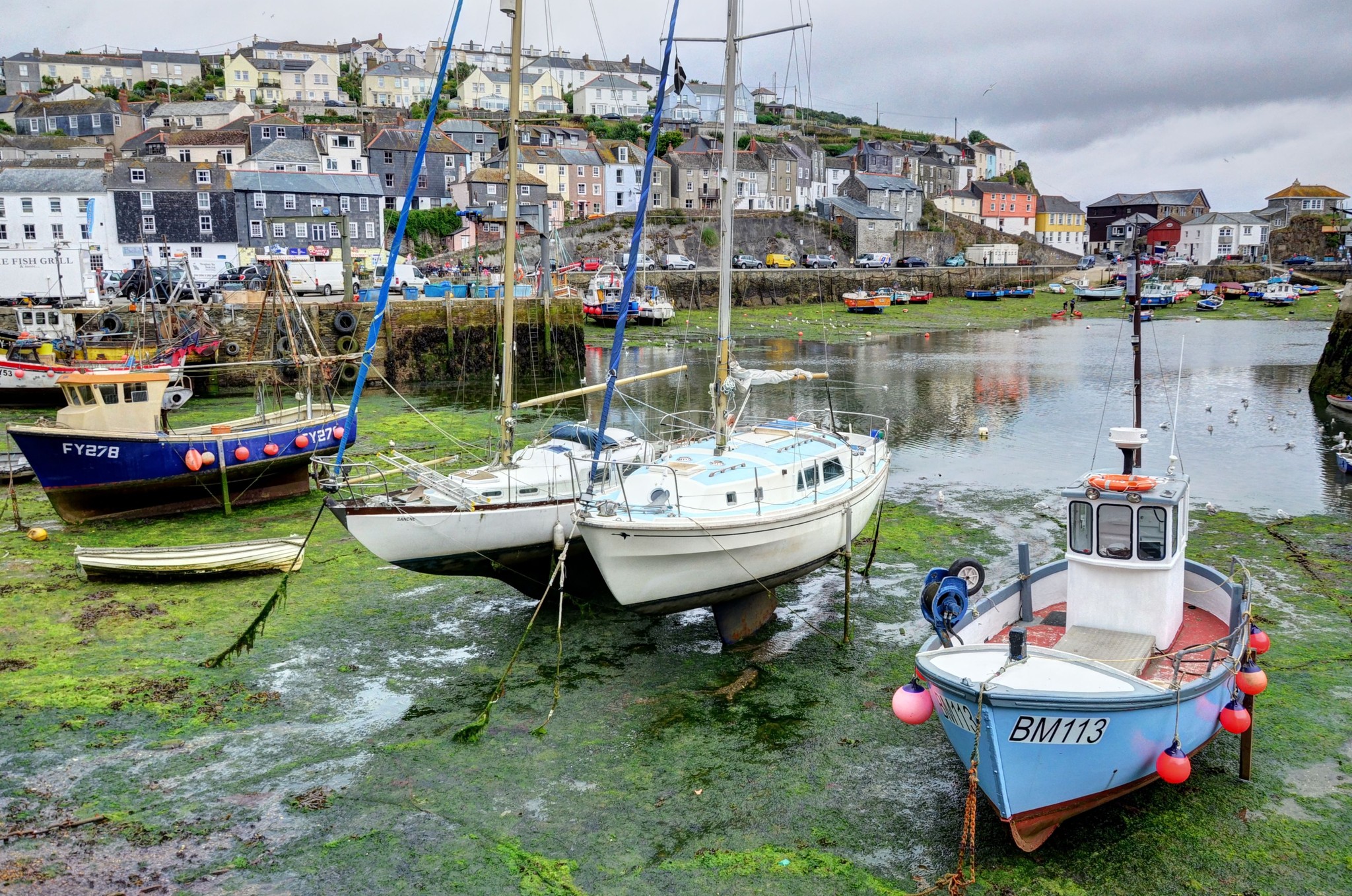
[0,0,892,615]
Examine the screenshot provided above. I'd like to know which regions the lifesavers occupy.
[1219,294,1224,299]
[276,336,299,355]
[334,311,356,334]
[33,305,52,309]
[336,335,358,355]
[617,280,619,287]
[1089,474,1157,491]
[915,287,918,290]
[98,313,124,334]
[276,314,300,335]
[478,256,483,263]
[514,268,523,280]
[649,301,652,306]
[17,336,37,339]
[672,299,674,306]
[226,342,240,355]
[340,363,358,383]
[858,286,861,290]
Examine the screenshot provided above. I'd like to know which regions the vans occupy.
[732,254,838,268]
[373,264,430,295]
[616,253,657,271]
[1076,255,1095,270]
[854,253,892,268]
[661,254,696,270]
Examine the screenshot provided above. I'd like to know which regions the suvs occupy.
[82,266,211,304]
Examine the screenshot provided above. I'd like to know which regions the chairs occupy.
[112,277,117,281]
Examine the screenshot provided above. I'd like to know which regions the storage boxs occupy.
[357,288,381,302]
[100,328,110,334]
[29,344,56,365]
[126,355,135,366]
[221,290,265,303]
[425,285,532,298]
[401,287,418,300]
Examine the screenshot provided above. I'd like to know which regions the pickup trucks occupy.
[218,262,276,291]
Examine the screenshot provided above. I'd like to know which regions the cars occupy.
[897,252,965,267]
[1281,256,1316,266]
[1099,249,1188,267]
[535,259,561,271]
[569,258,600,272]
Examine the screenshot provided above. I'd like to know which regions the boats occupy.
[1125,243,1344,323]
[1048,262,1154,299]
[963,267,1035,298]
[1051,310,1083,316]
[73,534,308,573]
[913,215,1251,852]
[1326,394,1352,473]
[841,266,933,308]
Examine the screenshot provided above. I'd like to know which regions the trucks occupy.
[0,247,91,305]
[288,261,361,295]
